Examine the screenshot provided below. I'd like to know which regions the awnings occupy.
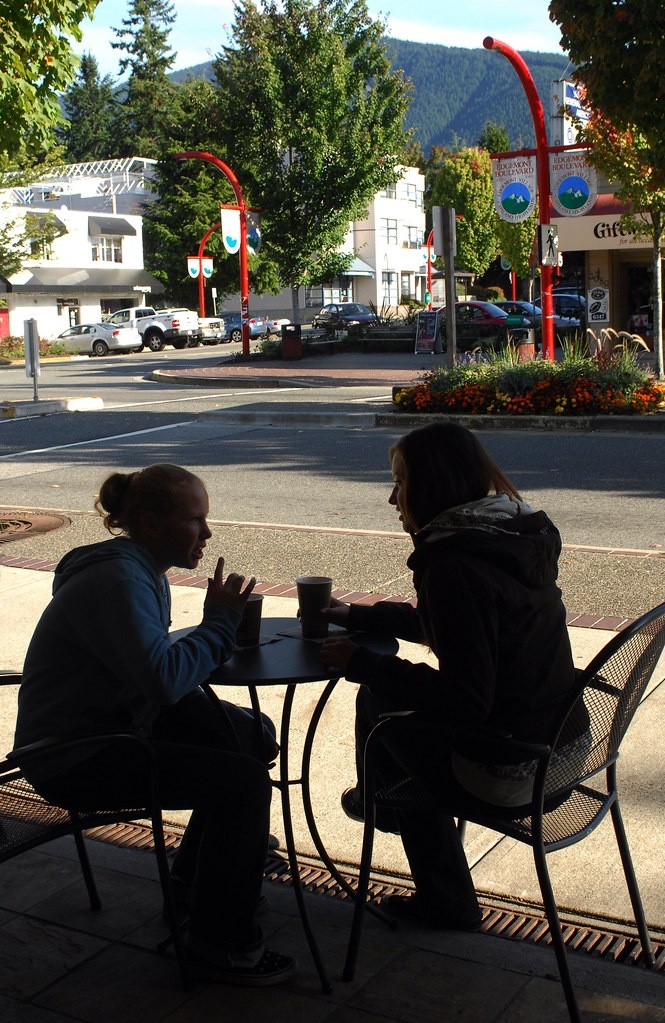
[25,211,66,233]
[88,216,137,236]
[339,253,375,278]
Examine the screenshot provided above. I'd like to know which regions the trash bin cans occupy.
[281,323,303,361]
[507,327,535,365]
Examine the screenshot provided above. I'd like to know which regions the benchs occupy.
[453,322,504,350]
[363,325,416,351]
[301,328,342,356]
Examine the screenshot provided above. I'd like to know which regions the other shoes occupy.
[341,789,401,832]
[176,893,268,931]
[382,895,481,927]
[186,947,298,987]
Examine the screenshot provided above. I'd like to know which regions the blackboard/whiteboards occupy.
[416,311,438,342]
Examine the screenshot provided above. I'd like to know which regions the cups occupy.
[296,577,333,638]
[233,593,264,647]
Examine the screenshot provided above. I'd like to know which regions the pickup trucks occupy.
[104,305,199,351]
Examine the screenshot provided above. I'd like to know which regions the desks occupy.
[169,616,407,997]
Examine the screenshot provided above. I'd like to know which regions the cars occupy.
[312,302,379,334]
[532,287,586,323]
[53,322,143,357]
[436,300,532,334]
[159,307,292,346]
[474,300,581,347]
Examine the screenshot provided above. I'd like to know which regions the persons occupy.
[295,421,593,932]
[11,462,300,989]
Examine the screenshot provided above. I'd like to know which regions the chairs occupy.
[504,306,509,313]
[0,669,174,921]
[338,602,665,1023]
[516,307,522,315]
